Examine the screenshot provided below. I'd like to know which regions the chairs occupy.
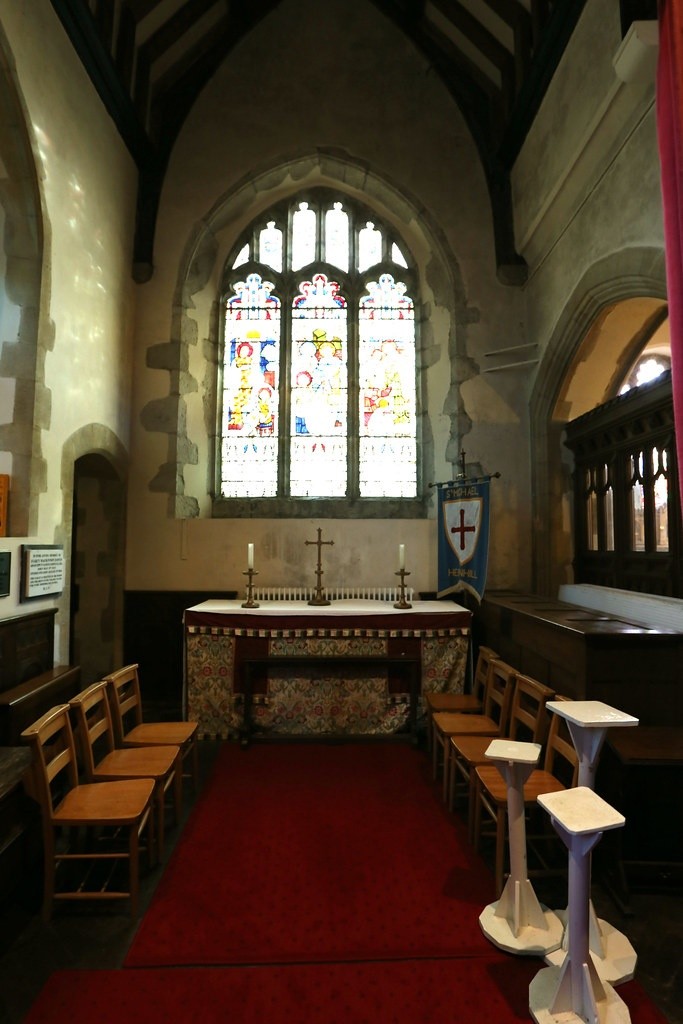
[21,663,198,928]
[424,643,578,903]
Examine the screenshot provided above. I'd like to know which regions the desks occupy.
[182,599,473,742]
[604,724,683,918]
[238,654,420,750]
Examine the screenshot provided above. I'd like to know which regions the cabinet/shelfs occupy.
[475,583,682,730]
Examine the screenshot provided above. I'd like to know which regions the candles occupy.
[248,542,253,570]
[400,545,405,570]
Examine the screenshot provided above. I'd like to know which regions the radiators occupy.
[246,587,415,601]
